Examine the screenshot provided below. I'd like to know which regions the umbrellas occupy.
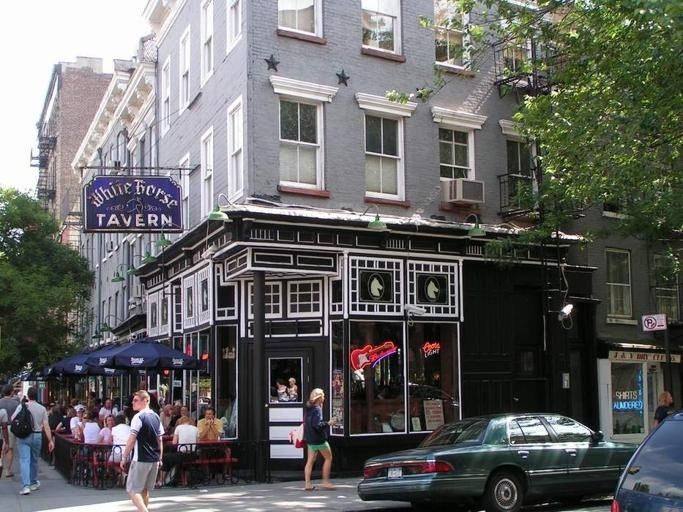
[84,338,202,389]
[21,346,123,410]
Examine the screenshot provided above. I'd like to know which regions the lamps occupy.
[111,264,131,283]
[142,241,158,264]
[155,222,190,247]
[127,255,145,275]
[462,213,486,236]
[362,204,387,230]
[207,193,237,221]
[100,314,124,332]
[92,323,114,338]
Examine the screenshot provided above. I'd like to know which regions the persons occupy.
[0,408,9,479]
[0,384,19,477]
[44,391,234,488]
[120,389,165,512]
[273,376,299,402]
[22,395,28,402]
[11,386,55,494]
[304,387,340,491]
[653,391,677,428]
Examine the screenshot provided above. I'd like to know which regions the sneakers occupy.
[18,479,40,494]
[5,471,13,477]
[117,473,128,487]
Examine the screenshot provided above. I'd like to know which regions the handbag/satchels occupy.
[290,424,304,448]
[10,408,35,438]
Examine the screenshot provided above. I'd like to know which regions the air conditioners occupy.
[447,177,486,205]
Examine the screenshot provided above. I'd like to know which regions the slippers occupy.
[306,483,337,491]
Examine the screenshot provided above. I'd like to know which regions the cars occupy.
[613,411,682,511]
[358,416,643,507]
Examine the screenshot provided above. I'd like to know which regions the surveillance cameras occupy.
[201,245,219,259]
[560,304,573,318]
[404,304,426,315]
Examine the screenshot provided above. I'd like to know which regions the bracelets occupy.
[122,456,127,461]
